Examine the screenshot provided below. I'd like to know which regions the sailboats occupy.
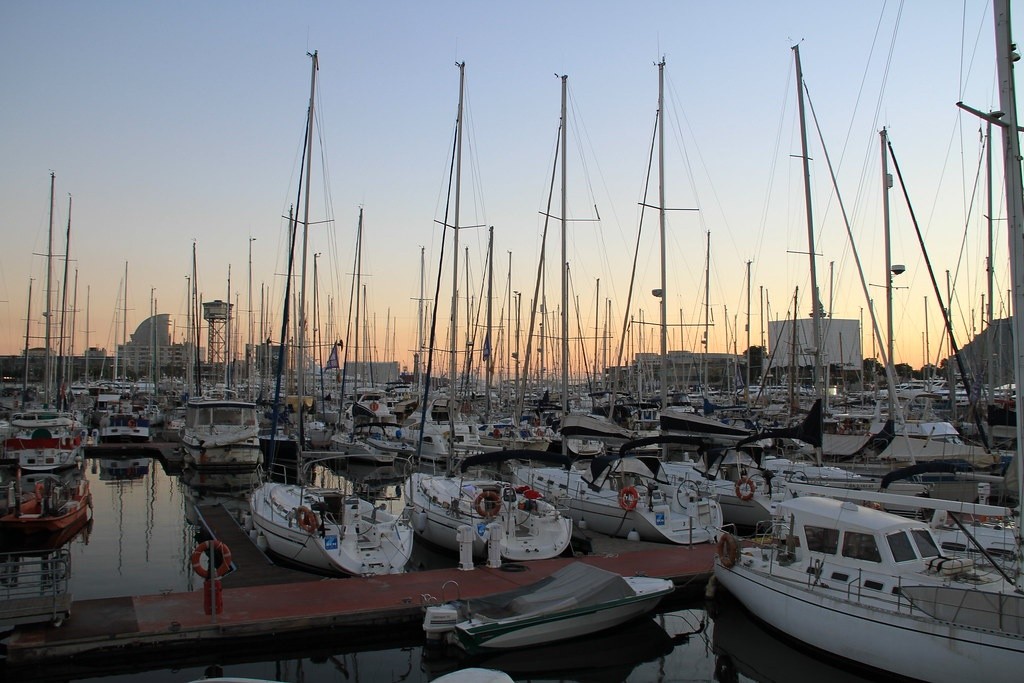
[0,0,1024,583]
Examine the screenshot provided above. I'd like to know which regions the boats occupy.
[419,613,676,672]
[0,526,95,558]
[417,560,675,653]
[710,497,1024,683]
[0,464,94,532]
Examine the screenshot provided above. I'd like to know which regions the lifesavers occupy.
[296,506,318,532]
[735,477,756,501]
[492,428,543,439]
[474,491,502,517]
[36,483,45,501]
[618,486,639,511]
[191,540,231,579]
[370,402,379,411]
